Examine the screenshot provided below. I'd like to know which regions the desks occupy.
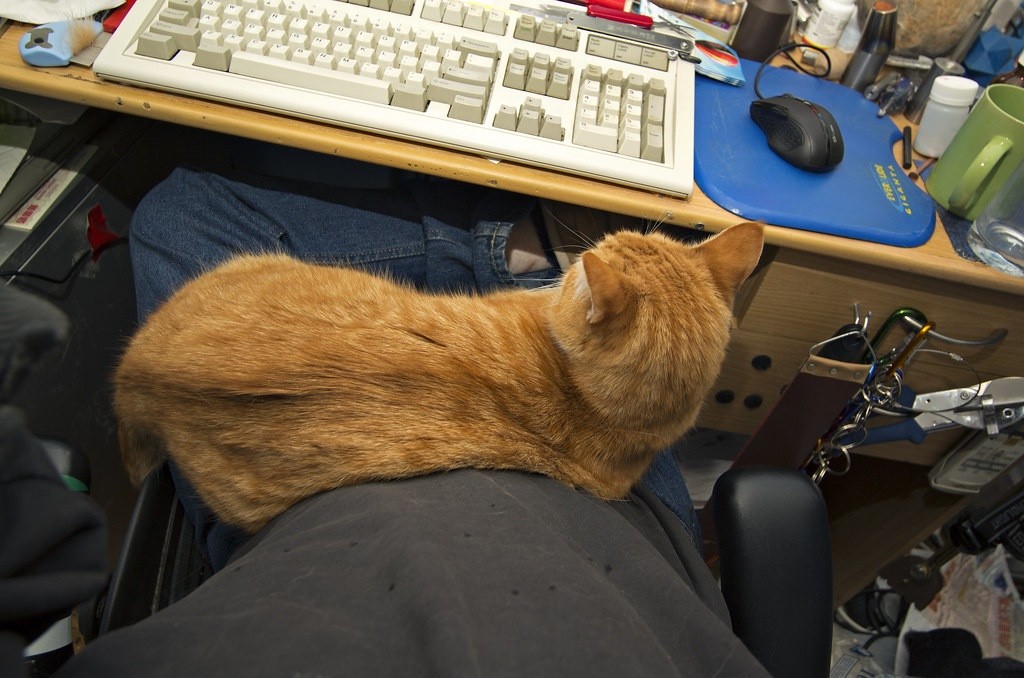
[0,0,1024,302]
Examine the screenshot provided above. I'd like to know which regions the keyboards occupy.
[92,0,702,200]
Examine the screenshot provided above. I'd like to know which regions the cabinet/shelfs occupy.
[667,247,1024,616]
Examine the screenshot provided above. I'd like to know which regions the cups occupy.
[966,152,1024,280]
[925,84,1024,222]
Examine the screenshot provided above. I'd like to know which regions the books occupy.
[638,0,746,90]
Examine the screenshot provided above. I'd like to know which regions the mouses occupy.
[750,93,844,172]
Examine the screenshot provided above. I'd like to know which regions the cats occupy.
[109,223,764,531]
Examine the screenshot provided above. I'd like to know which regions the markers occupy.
[902,126,913,170]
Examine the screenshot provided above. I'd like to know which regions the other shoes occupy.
[540,198,637,276]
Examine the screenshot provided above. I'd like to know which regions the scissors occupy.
[587,4,699,39]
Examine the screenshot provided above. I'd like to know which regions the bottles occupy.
[728,0,795,64]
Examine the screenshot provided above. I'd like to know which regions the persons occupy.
[1,169,778,678]
